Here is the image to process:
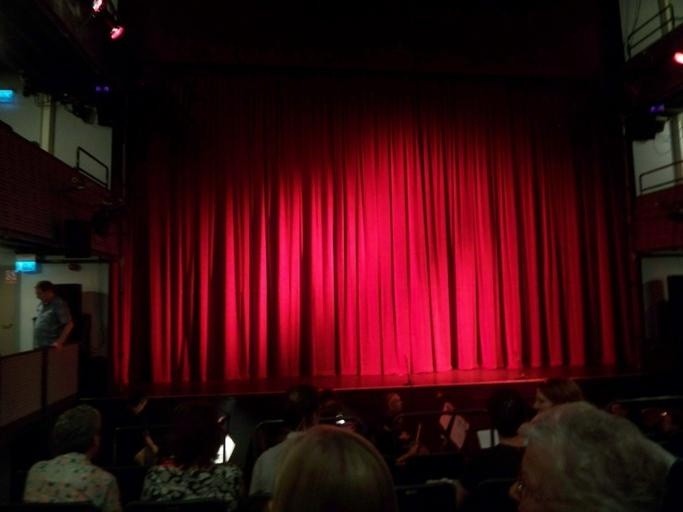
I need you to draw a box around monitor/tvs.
[211,432,238,464]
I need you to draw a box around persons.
[15,377,683,510]
[31,280,75,351]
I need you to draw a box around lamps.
[616,93,682,143]
[81,0,123,40]
